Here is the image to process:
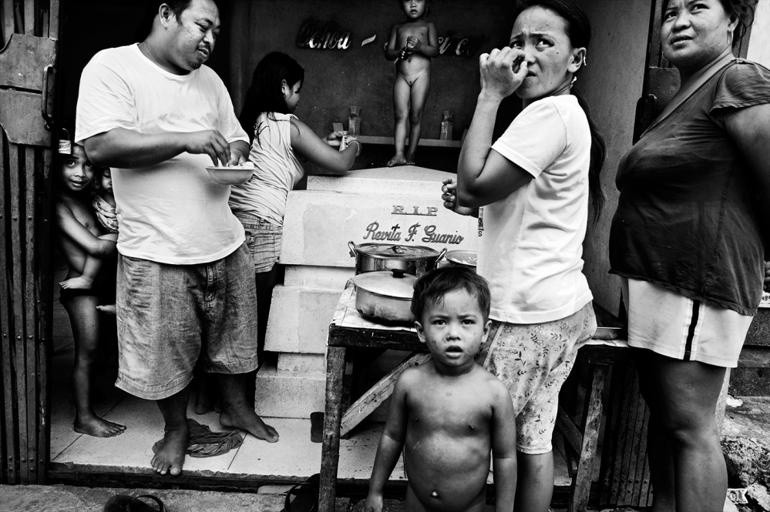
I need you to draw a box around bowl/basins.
[209,165,253,182]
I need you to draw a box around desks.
[318,279,635,510]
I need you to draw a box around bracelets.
[347,140,361,157]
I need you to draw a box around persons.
[382,0,442,167]
[57,165,119,315]
[73,0,279,473]
[363,262,518,512]
[439,2,597,511]
[191,48,363,416]
[53,137,128,440]
[606,1,770,512]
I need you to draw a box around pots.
[352,272,429,324]
[348,234,443,275]
